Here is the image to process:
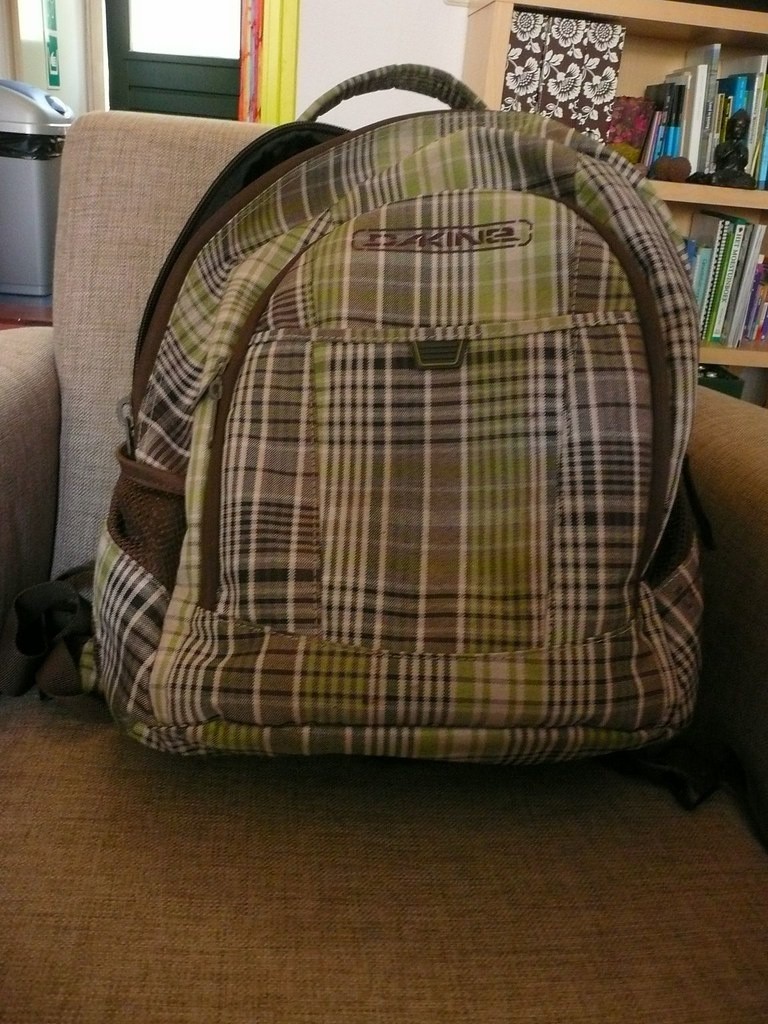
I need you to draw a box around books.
[638,44,768,189]
[681,209,768,349]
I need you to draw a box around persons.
[710,108,756,188]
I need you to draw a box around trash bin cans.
[0,76,73,297]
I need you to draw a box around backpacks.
[80,63,707,770]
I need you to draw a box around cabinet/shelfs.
[462,0,768,410]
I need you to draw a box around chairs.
[0,108,768,1022]
